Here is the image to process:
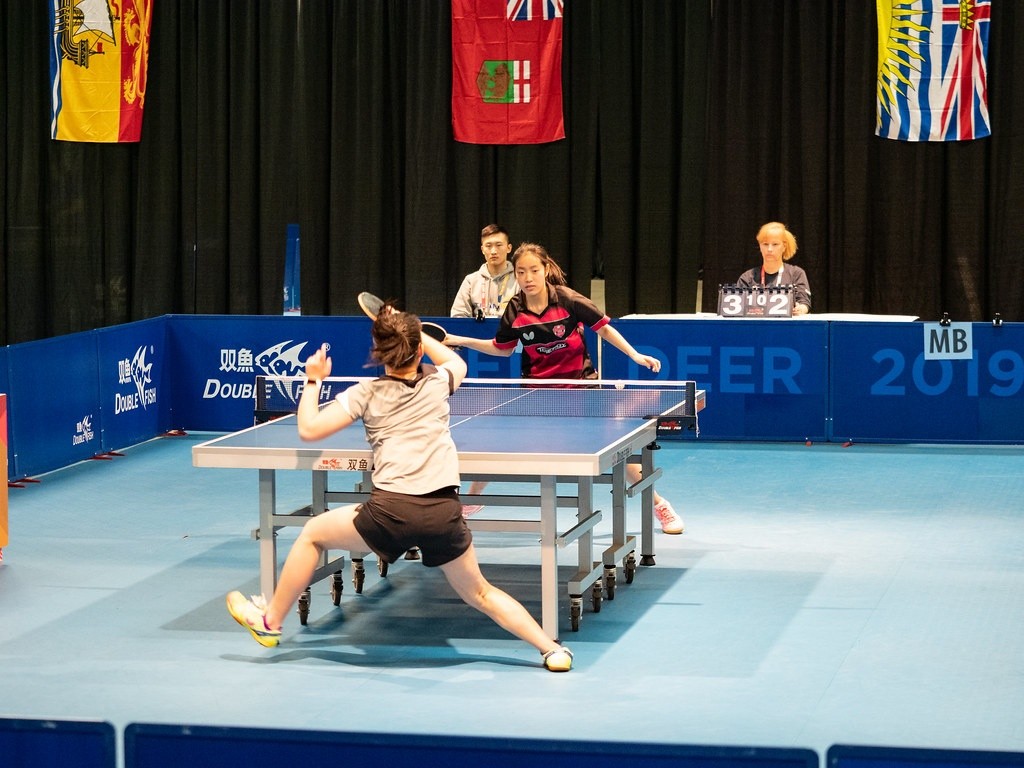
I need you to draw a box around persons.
[443,242,685,534]
[226,306,574,673]
[450,226,523,319]
[737,223,812,314]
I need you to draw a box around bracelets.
[303,378,322,388]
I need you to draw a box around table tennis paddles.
[358,292,384,320]
[420,321,463,350]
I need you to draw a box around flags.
[451,0,567,145]
[46,0,151,143]
[877,0,991,141]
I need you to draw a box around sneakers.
[541,648,573,670]
[654,497,684,533]
[461,504,483,518]
[226,590,282,648]
